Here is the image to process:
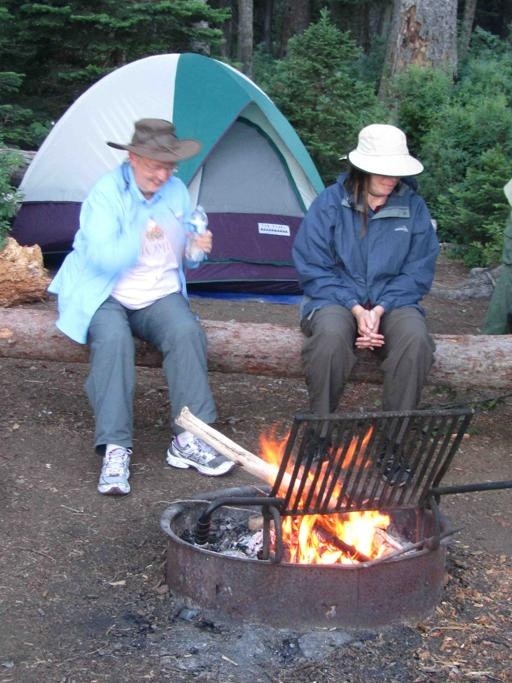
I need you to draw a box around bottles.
[185,202,208,262]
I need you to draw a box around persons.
[292,124,440,489]
[47,117,236,497]
[484,178,512,338]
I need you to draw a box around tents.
[12,52,324,306]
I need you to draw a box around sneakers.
[296,435,412,487]
[96,448,134,496]
[165,434,235,477]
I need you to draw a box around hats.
[337,123,425,178]
[107,118,201,164]
